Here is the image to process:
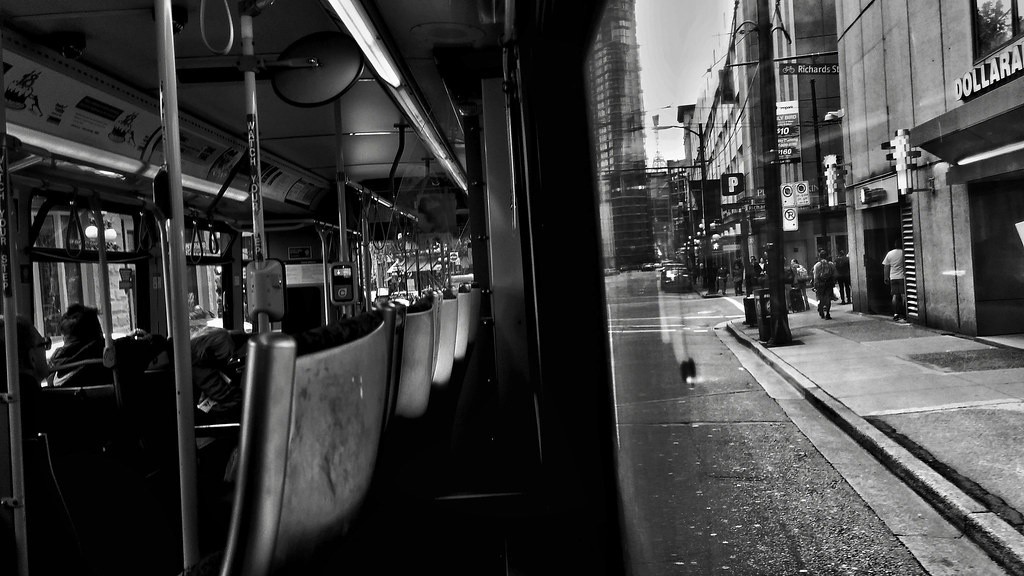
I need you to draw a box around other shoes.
[826,314,832,319]
[848,301,851,303]
[842,301,845,304]
[894,315,900,321]
[821,314,825,318]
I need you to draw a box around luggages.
[790,284,805,313]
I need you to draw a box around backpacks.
[820,261,833,282]
[835,257,849,273]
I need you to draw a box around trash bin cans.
[742,297,758,325]
[752,288,772,342]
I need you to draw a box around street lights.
[651,173,696,284]
[656,125,716,294]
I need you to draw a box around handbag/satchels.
[796,265,808,280]
[734,274,742,282]
[765,274,768,280]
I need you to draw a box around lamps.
[894,129,935,197]
[824,163,847,207]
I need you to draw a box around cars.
[654,261,692,293]
[605,262,656,275]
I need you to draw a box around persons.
[0,314,50,397]
[881,239,904,321]
[187,328,248,485]
[712,246,851,321]
[43,305,106,391]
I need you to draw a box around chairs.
[0,283,483,576]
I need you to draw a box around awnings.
[909,76,1024,164]
